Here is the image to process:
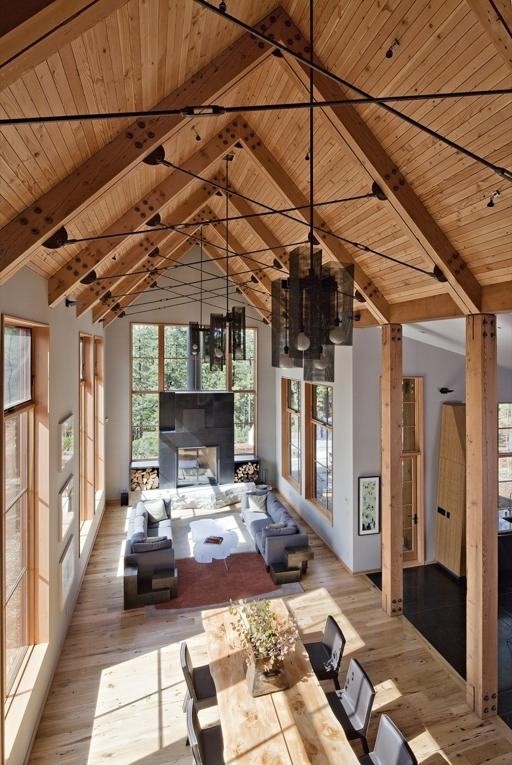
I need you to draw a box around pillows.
[247,494,267,513]
[145,500,168,525]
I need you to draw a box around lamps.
[272,279,303,368]
[209,313,223,372]
[289,246,320,359]
[203,324,211,364]
[231,306,246,360]
[320,260,355,346]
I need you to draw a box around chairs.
[304,616,346,691]
[326,658,376,753]
[357,714,416,765]
[179,642,217,713]
[184,699,224,765]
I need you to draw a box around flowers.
[223,601,299,667]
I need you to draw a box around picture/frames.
[357,475,382,537]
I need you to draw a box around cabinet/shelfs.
[159,390,235,488]
[401,376,424,455]
[434,403,467,578]
[401,458,426,569]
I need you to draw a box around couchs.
[124,502,176,573]
[240,490,310,573]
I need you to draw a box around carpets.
[155,552,275,609]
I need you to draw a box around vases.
[245,654,290,697]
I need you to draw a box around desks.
[202,601,360,765]
